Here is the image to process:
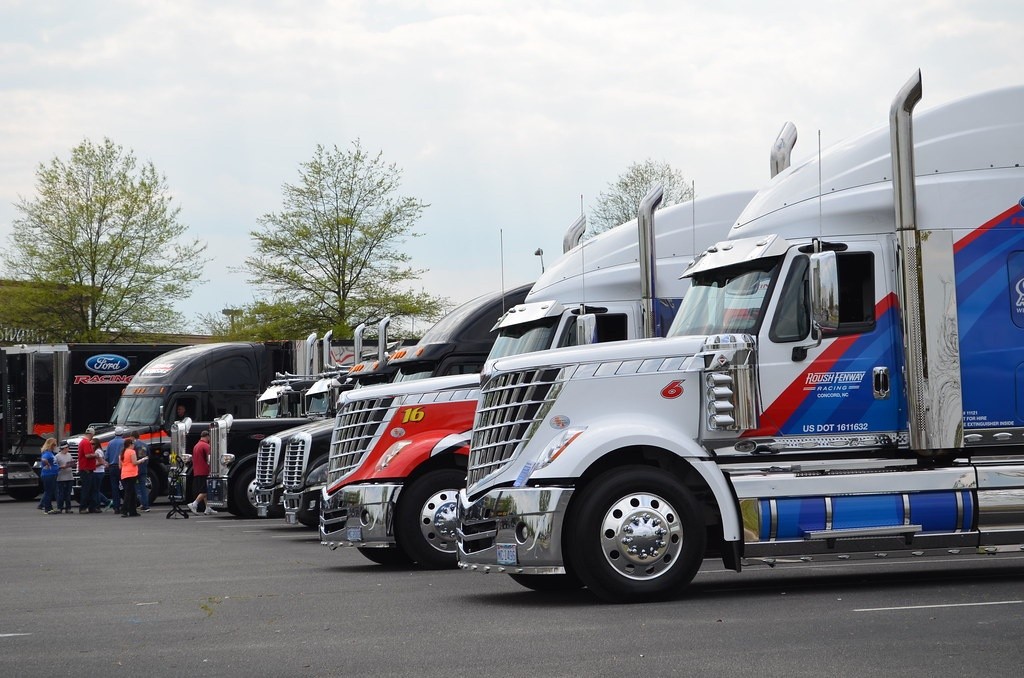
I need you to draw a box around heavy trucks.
[168,327,426,517]
[33,338,431,513]
[244,67,1024,603]
[0,343,193,504]
[206,280,539,522]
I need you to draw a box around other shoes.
[121,512,129,517]
[35,505,45,511]
[44,510,50,514]
[79,507,88,513]
[141,506,151,512]
[129,513,141,517]
[65,508,73,514]
[88,508,102,513]
[104,500,113,511]
[137,505,143,509]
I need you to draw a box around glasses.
[91,443,95,445]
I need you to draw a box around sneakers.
[204,509,217,515]
[188,503,199,515]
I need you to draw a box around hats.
[86,428,95,434]
[59,440,69,448]
[115,427,124,435]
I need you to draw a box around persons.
[187,430,218,515]
[38,428,150,518]
[175,406,185,420]
